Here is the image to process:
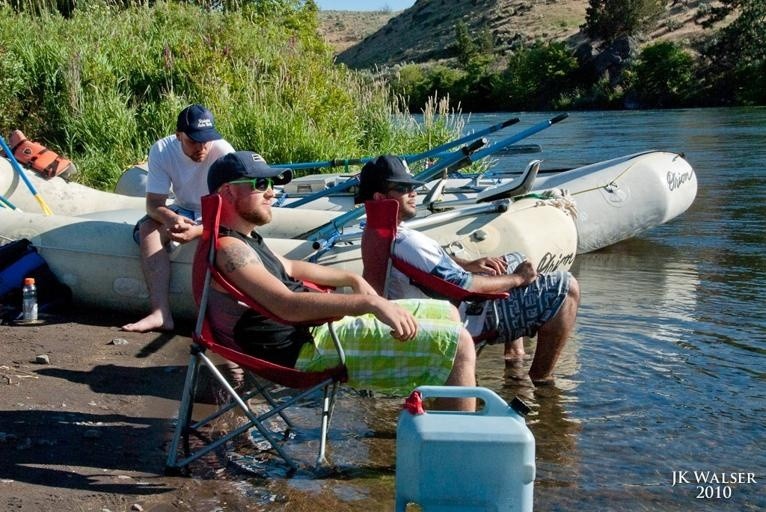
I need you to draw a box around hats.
[358,154,426,191]
[206,151,293,195]
[175,104,222,143]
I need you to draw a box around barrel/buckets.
[396,385,536,512]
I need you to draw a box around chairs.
[167,192,345,467]
[362,198,511,302]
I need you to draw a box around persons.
[200,149,479,412]
[120,102,238,333]
[355,152,584,384]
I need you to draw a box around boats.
[0,149,698,318]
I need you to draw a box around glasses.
[218,177,276,192]
[391,187,419,193]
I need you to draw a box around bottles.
[23,278,39,321]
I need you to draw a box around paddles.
[0,137,53,216]
